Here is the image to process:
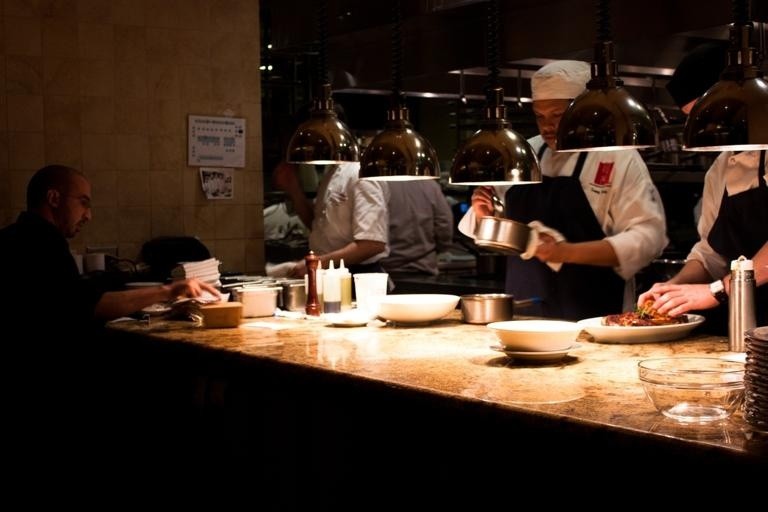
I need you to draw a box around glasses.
[64,195,93,209]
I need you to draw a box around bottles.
[305,251,352,316]
[728,255,758,354]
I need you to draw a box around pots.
[456,294,542,325]
[475,215,532,258]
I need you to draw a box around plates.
[577,313,706,344]
[744,326,767,428]
[140,307,172,317]
[488,343,583,363]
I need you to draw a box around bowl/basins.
[370,293,461,324]
[487,320,583,350]
[638,358,746,422]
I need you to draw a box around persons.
[205,172,232,197]
[262,57,768,336]
[1,164,221,511]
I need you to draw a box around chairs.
[138,235,211,263]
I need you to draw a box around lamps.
[287,1,362,166]
[680,2,767,154]
[446,1,543,186]
[554,0,656,154]
[357,0,441,182]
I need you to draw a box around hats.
[531,60,591,103]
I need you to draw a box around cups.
[355,272,389,314]
